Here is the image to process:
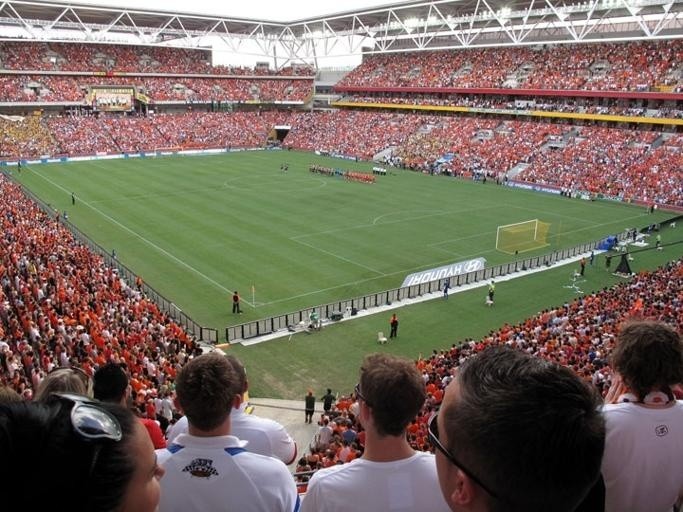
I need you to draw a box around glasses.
[353,384,374,411]
[50,394,121,472]
[425,412,495,502]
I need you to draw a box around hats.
[92,361,128,395]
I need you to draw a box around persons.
[166,355,299,465]
[591,321,682,512]
[0,386,165,510]
[1,35,683,215]
[232,290,240,313]
[0,181,203,451]
[427,348,606,511]
[154,351,300,512]
[294,351,452,511]
[294,224,683,490]
